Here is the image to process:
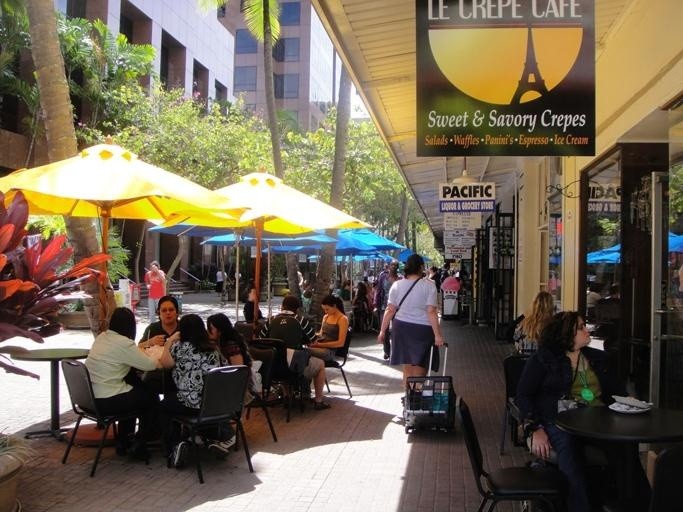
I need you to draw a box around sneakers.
[168,440,189,468]
[128,442,150,460]
[207,441,230,459]
[220,434,238,448]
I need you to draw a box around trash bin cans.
[169,292,183,312]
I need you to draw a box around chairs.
[311,327,351,400]
[458,354,683,512]
[252,338,305,424]
[233,344,279,449]
[594,301,620,329]
[61,359,149,477]
[169,369,249,484]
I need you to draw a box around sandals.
[315,400,330,409]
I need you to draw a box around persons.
[511,311,651,512]
[432,263,468,318]
[548,272,561,300]
[146,260,401,409]
[587,275,620,324]
[379,254,444,420]
[85,297,248,466]
[514,290,560,350]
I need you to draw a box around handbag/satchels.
[555,392,588,413]
[519,456,563,511]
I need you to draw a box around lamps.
[453,156,480,183]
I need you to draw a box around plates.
[608,395,652,414]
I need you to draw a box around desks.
[10,348,90,442]
